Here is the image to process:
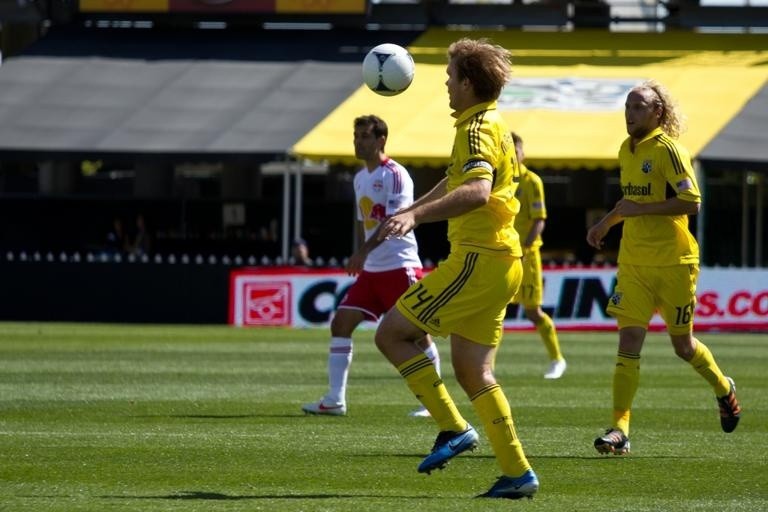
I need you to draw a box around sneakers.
[408,401,432,418]
[301,395,348,416]
[716,376,741,433]
[543,358,567,379]
[470,468,540,501]
[594,427,631,456]
[417,422,480,476]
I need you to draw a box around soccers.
[361,42,414,96]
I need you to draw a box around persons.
[375,36,540,500]
[511,132,566,379]
[587,85,740,454]
[304,116,441,417]
